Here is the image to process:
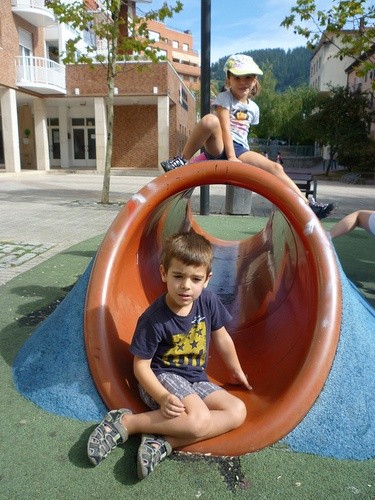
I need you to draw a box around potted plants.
[23,129,31,144]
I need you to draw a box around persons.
[87,233,254,480]
[327,209,375,238]
[160,54,335,221]
[260,151,284,165]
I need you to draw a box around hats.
[222,53,264,78]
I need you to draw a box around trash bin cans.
[225,186,252,215]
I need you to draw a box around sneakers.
[308,197,335,220]
[160,156,189,172]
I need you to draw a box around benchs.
[284,168,317,201]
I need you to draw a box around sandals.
[137,432,173,479]
[88,408,132,466]
